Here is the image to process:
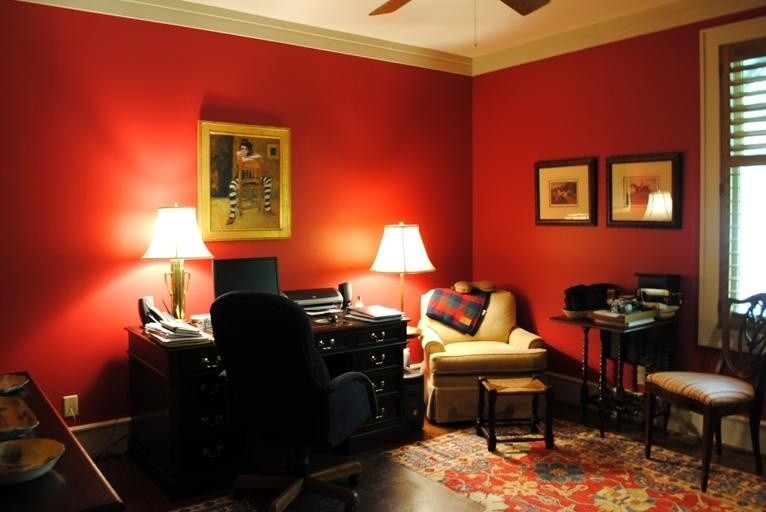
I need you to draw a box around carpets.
[175,415,766,512]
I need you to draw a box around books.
[144,317,215,347]
[345,304,404,323]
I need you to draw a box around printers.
[281,287,344,316]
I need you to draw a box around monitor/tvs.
[212,256,279,299]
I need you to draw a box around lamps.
[370,222,435,314]
[140,204,214,318]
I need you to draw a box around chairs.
[644,293,766,492]
[210,289,379,512]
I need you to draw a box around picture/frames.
[534,157,597,226]
[605,152,682,229]
[196,120,292,243]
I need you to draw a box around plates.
[0,438,65,481]
[0,374,30,393]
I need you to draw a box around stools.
[475,372,553,451]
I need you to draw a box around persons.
[223,136,274,226]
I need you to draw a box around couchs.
[417,288,548,422]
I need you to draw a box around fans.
[367,0,549,16]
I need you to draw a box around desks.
[124,307,411,502]
[0,371,121,512]
[548,315,681,439]
